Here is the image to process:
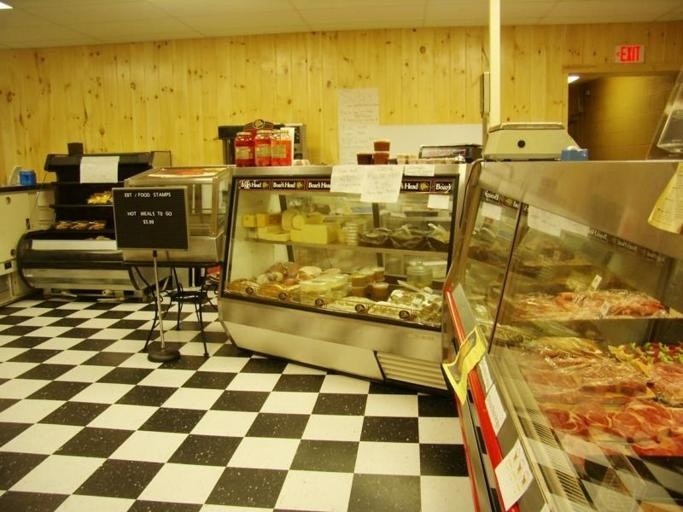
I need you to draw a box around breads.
[228,259,341,304]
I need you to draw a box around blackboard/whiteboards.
[112,186,189,251]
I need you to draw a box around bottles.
[233,128,293,167]
[405,258,433,289]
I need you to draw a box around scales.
[481,122,580,161]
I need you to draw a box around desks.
[124,257,224,357]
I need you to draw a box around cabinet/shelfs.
[215,163,472,393]
[16,149,174,304]
[436,153,682,512]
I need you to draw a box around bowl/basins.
[358,230,449,250]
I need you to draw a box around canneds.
[233,130,292,167]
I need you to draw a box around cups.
[18,170,35,186]
